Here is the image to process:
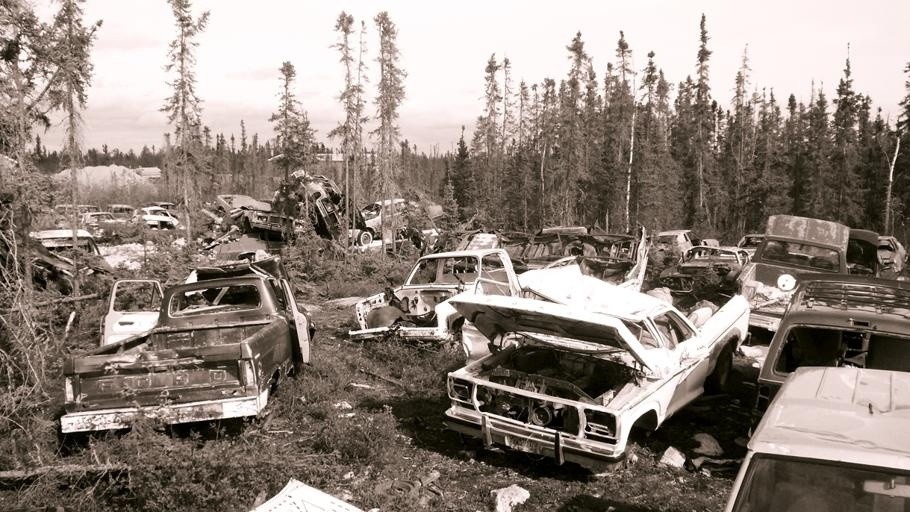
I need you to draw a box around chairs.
[765,245,788,261]
[810,255,832,271]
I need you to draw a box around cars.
[347,212,910,478]
[23,171,444,296]
[720,361,910,510]
[60,249,318,433]
